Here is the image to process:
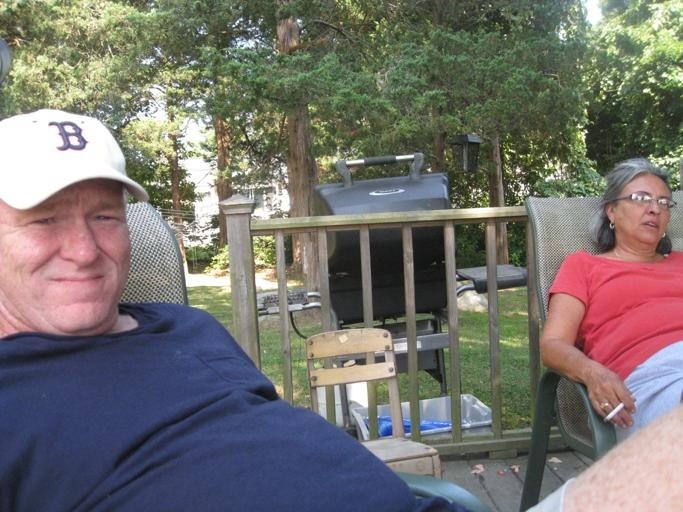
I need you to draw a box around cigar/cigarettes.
[601,401,625,424]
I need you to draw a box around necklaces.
[611,246,662,264]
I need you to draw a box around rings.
[597,401,610,411]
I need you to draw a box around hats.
[1,108,151,212]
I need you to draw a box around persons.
[538,156,682,446]
[0,108,682,512]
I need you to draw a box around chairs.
[305,328,442,480]
[518,191,683,512]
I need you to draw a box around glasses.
[606,193,676,209]
